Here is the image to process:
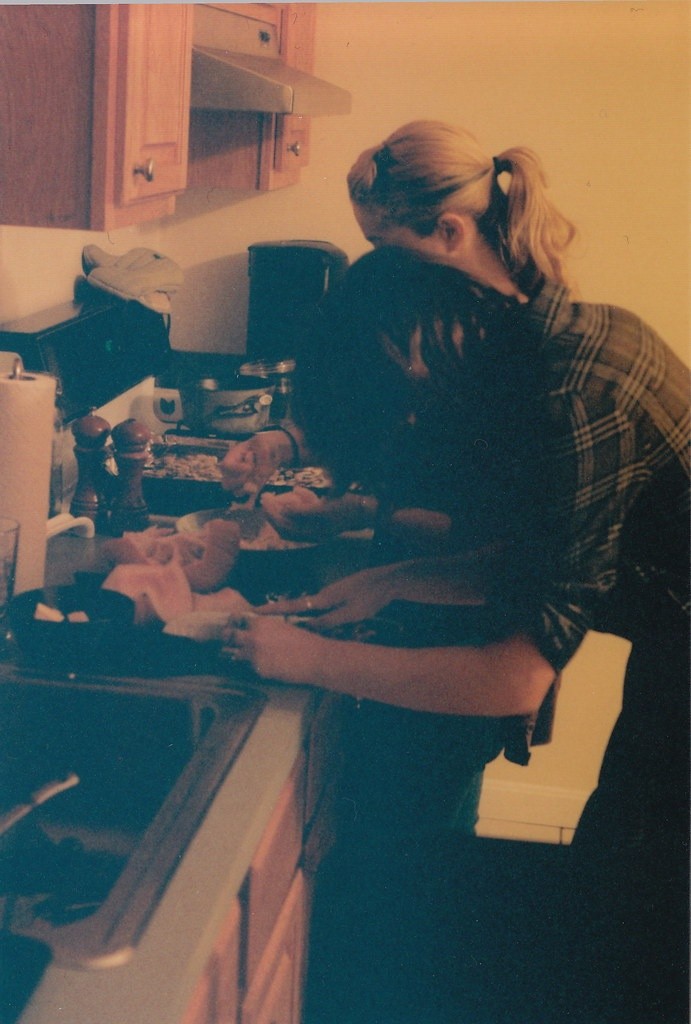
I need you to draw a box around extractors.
[190,4,352,115]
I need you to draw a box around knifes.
[161,610,405,646]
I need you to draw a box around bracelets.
[260,425,301,469]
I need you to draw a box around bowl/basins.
[175,508,345,580]
[241,360,299,396]
[7,584,136,668]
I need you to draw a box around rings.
[305,598,313,609]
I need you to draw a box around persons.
[219,121,690,1024]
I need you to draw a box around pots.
[183,370,277,434]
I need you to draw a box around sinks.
[0,672,271,970]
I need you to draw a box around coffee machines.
[246,239,350,356]
